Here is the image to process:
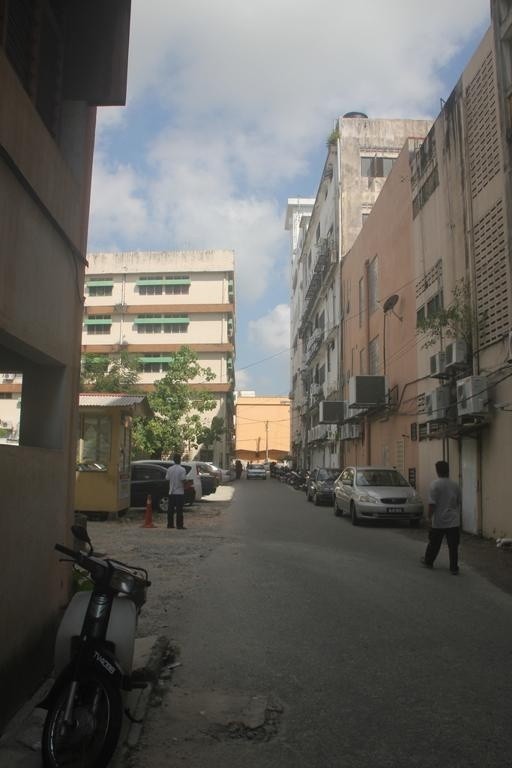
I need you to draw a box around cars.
[262,461,281,472]
[304,463,345,507]
[332,464,424,527]
[129,455,237,514]
[247,461,269,481]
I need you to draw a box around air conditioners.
[444,343,466,368]
[430,352,452,377]
[319,401,343,424]
[417,388,450,425]
[313,329,322,337]
[348,376,384,408]
[311,384,321,396]
[456,375,488,417]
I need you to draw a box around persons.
[165,455,188,529]
[421,461,463,575]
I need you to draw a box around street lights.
[264,418,273,462]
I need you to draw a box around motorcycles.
[34,516,161,767]
[272,463,313,494]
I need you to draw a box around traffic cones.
[140,494,157,528]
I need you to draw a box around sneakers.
[420,555,435,568]
[166,524,187,529]
[450,565,461,575]
